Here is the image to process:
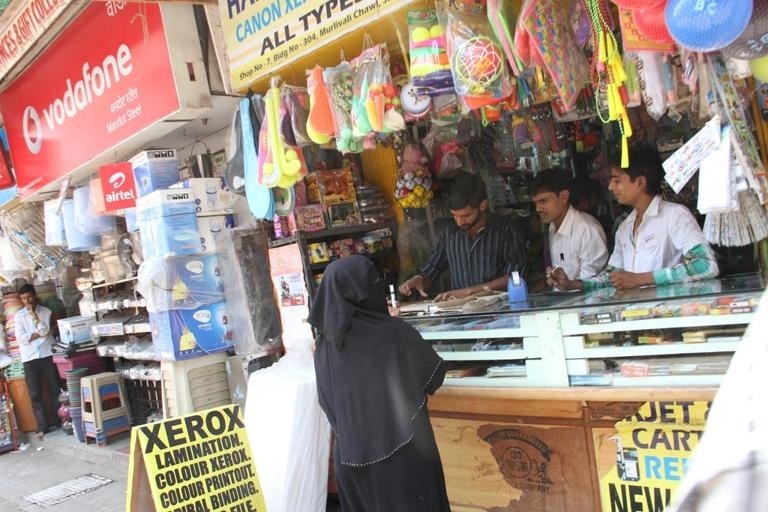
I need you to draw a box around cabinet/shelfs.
[91,275,161,422]
[268,167,764,512]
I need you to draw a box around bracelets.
[412,275,427,280]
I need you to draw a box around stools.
[80,371,135,447]
[161,351,233,420]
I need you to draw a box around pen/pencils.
[389,284,396,308]
[546,259,563,277]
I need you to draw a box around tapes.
[511,271,520,287]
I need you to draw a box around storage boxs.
[57,315,96,346]
[137,179,235,358]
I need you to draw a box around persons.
[171,274,191,304]
[399,170,527,304]
[541,143,721,293]
[179,325,197,352]
[304,253,457,512]
[14,283,65,441]
[530,166,610,293]
